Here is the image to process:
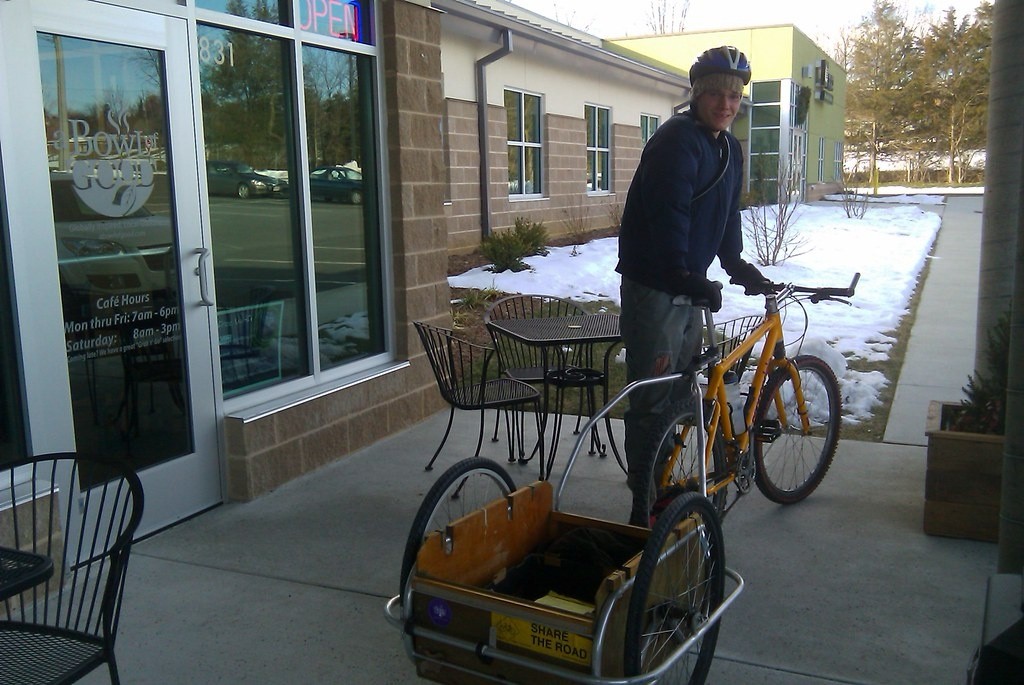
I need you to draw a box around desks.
[485,313,629,480]
[0,545,53,603]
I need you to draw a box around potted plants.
[923,295,1014,543]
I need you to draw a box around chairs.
[412,320,545,503]
[61,283,277,459]
[484,295,594,464]
[676,314,764,426]
[0,453,145,685]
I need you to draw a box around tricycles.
[384,272,861,685]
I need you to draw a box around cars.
[52,171,178,329]
[310,166,363,204]
[205,160,284,199]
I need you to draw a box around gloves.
[688,275,723,312]
[730,264,770,295]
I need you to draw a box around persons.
[614,46,769,529]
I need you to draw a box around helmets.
[689,46,752,87]
